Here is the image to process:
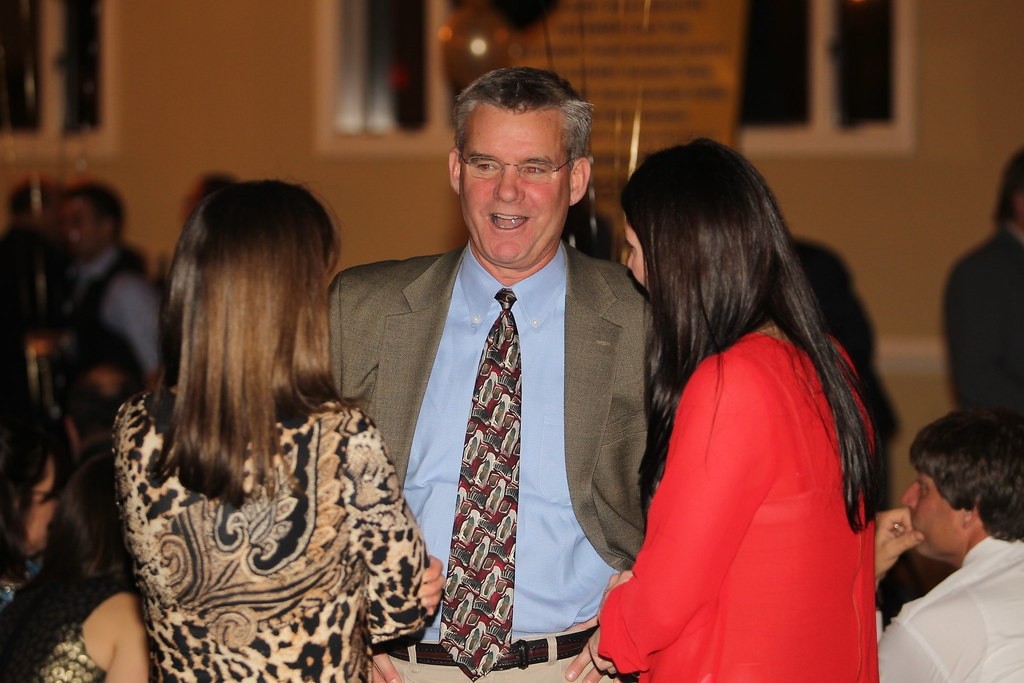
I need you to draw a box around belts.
[384,627,600,671]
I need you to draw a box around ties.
[439,288,523,680]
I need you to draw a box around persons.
[0,171,235,683]
[790,142,1024,683]
[329,65,654,683]
[589,136,880,683]
[111,178,446,683]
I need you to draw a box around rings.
[889,523,901,532]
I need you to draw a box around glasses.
[459,152,577,185]
[27,487,56,505]
